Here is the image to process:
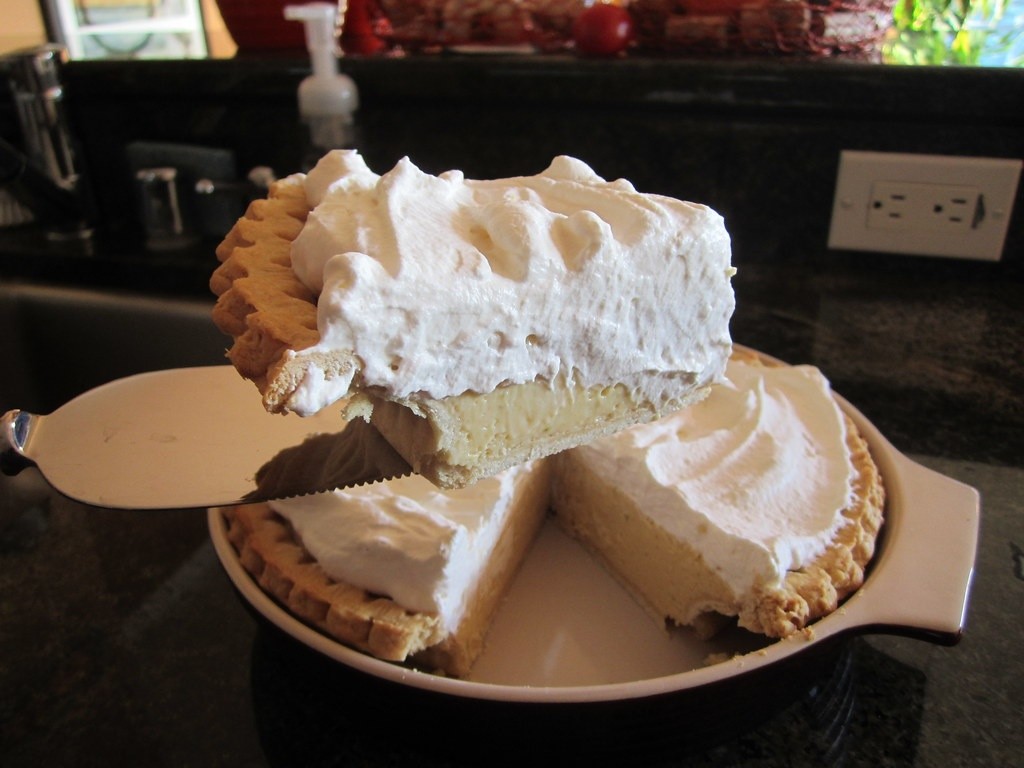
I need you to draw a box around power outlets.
[827,151,1023,262]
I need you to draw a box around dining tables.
[0,446,1024,768]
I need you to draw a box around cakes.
[213,147,739,480]
[221,346,889,679]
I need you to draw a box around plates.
[206,339,981,705]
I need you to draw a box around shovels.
[0,363,433,511]
